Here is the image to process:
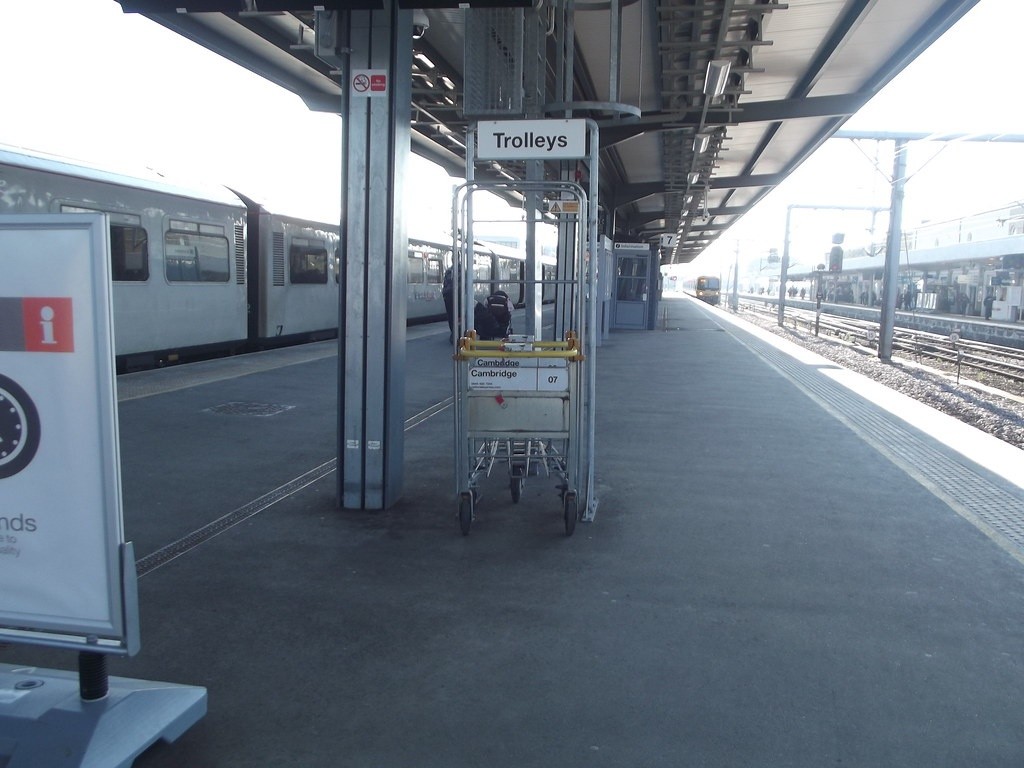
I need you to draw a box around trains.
[0,148,556,374]
[683,275,721,305]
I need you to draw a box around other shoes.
[449,338,453,344]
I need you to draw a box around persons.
[958,293,970,316]
[800,288,805,299]
[823,289,827,300]
[897,289,917,310]
[758,287,770,295]
[443,254,467,344]
[860,291,876,306]
[788,286,798,297]
[474,291,514,338]
[750,287,754,293]
[984,294,993,320]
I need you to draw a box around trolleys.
[457,328,584,536]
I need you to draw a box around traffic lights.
[828,246,842,272]
